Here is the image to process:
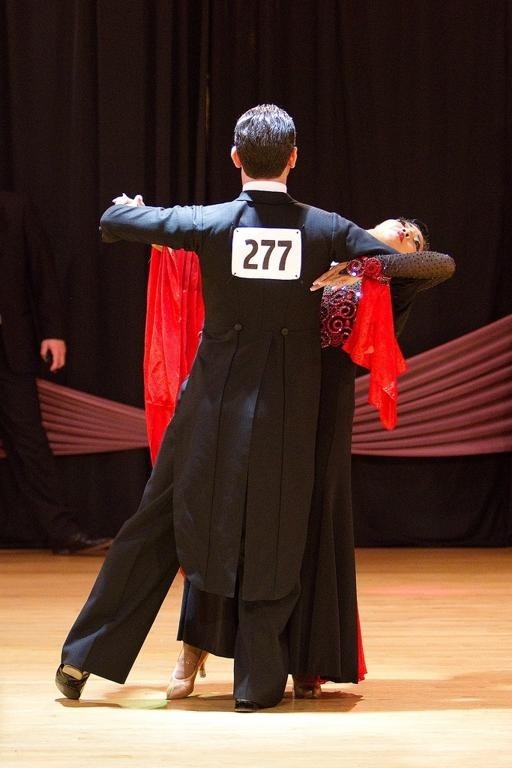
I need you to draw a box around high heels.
[166,647,210,699]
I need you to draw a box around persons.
[55,105,405,712]
[114,194,456,700]
[0,190,113,555]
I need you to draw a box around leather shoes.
[56,532,115,555]
[289,677,329,700]
[53,659,89,701]
[231,692,266,711]
